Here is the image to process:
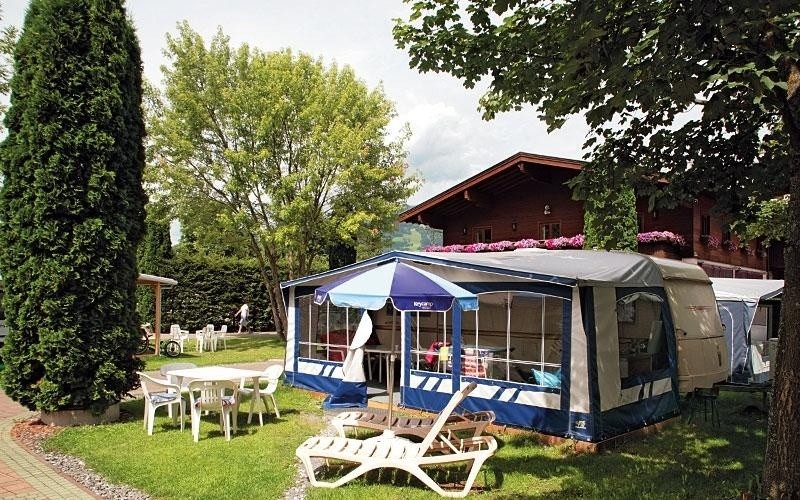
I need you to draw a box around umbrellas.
[312,257,479,432]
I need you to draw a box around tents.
[710,278,784,385]
[280,250,729,443]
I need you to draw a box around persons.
[234,301,251,334]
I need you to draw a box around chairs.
[170,324,190,353]
[294,381,499,499]
[136,359,285,443]
[196,323,228,353]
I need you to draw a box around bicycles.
[134,329,181,357]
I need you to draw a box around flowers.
[700,234,756,257]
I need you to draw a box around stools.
[687,386,721,430]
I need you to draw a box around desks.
[364,344,514,394]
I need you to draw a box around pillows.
[530,367,561,387]
